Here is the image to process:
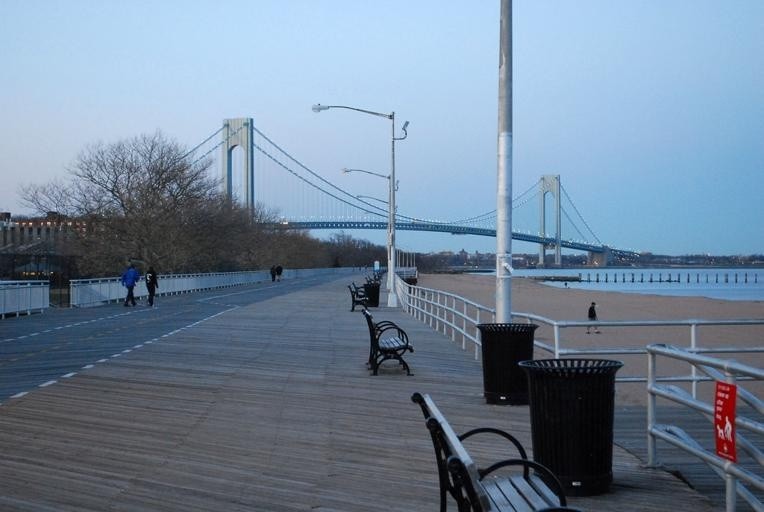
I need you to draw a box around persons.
[270,264,276,283]
[145,266,159,306]
[121,264,141,307]
[275,264,283,282]
[585,301,601,334]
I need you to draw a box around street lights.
[309,102,411,307]
[342,166,397,288]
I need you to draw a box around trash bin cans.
[475,323,540,404]
[518,358,624,497]
[364,283,381,306]
[405,278,417,293]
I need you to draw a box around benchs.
[361,309,414,376]
[347,282,369,312]
[411,392,586,512]
[365,270,386,284]
